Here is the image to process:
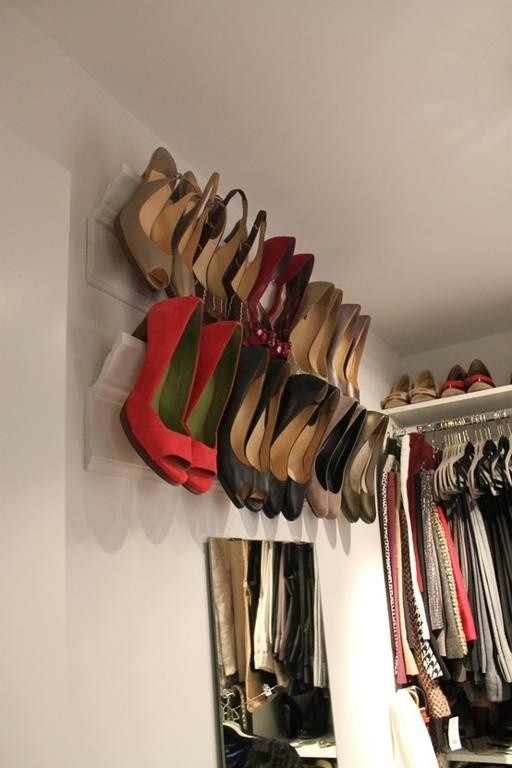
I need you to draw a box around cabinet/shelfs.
[86,165,339,498]
[373,384,511,768]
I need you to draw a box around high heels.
[298,690,330,740]
[111,146,390,525]
[281,693,299,738]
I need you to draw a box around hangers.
[432,409,511,503]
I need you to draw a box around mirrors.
[205,537,338,768]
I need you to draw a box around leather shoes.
[440,363,467,396]
[410,369,439,403]
[383,373,412,407]
[466,358,496,391]
